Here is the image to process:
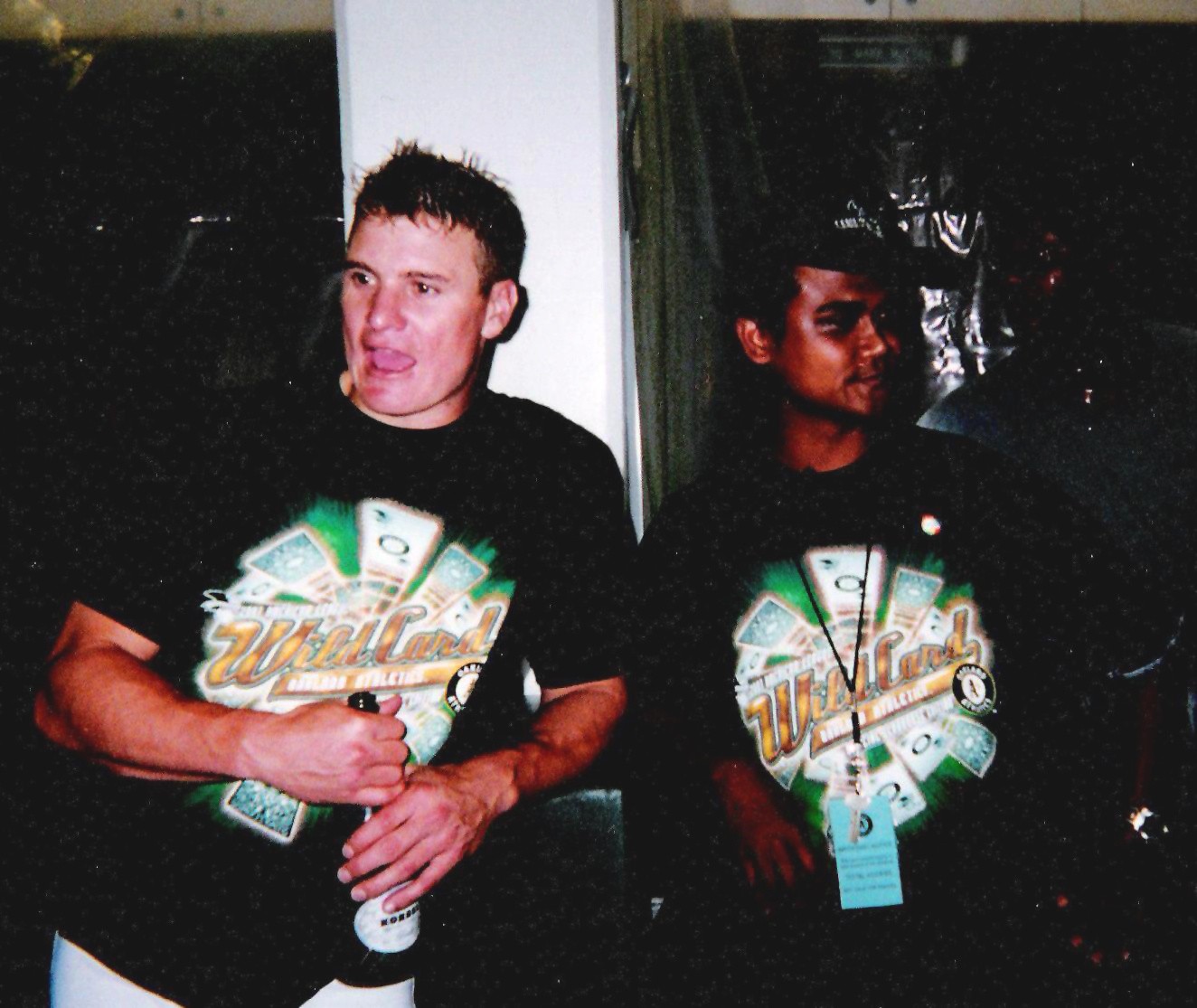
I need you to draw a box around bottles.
[328,692,428,986]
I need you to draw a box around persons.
[33,133,639,1008]
[639,223,1045,1008]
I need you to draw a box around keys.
[842,791,870,843]
